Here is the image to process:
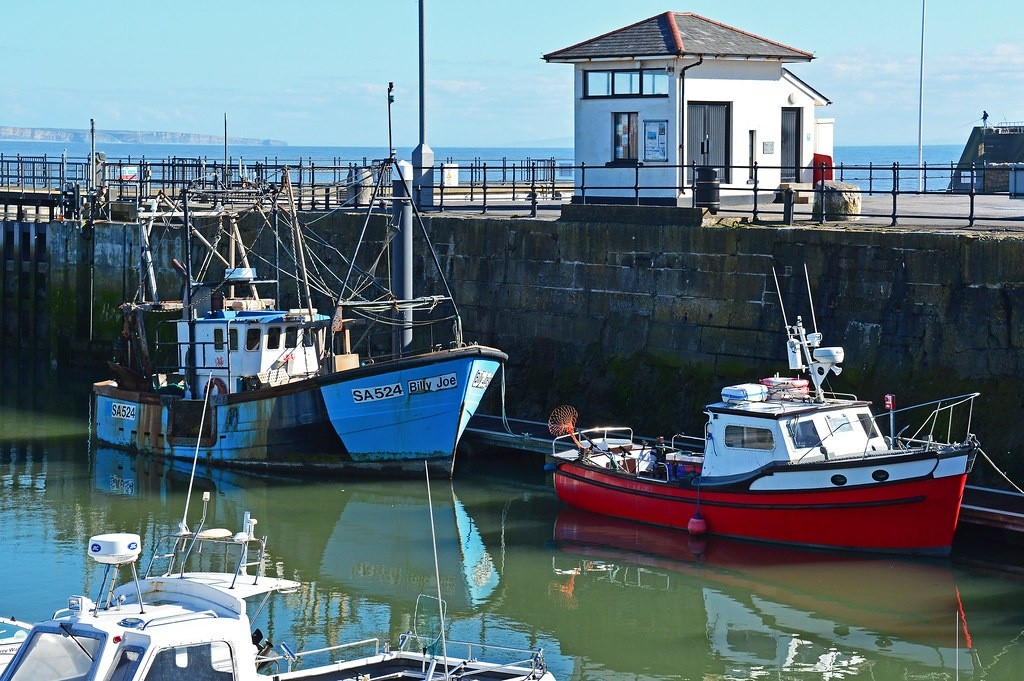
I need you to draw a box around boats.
[1,512,564,681]
[91,263,513,486]
[548,327,978,567]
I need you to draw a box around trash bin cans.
[694,166,720,216]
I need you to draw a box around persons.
[981,109,990,129]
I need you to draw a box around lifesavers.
[203,378,227,400]
[763,377,809,388]
[768,387,810,400]
